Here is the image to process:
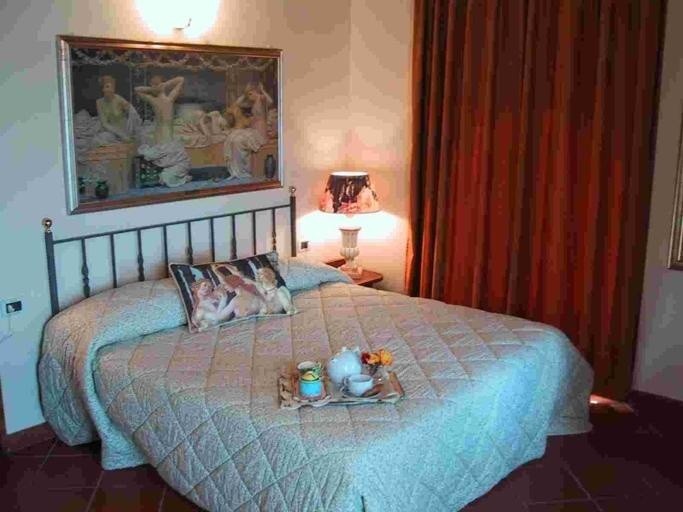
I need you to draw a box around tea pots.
[325,345,364,387]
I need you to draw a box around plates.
[340,385,382,399]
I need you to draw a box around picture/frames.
[55,34,284,215]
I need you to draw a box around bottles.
[299,373,321,397]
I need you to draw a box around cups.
[297,360,320,372]
[342,374,372,396]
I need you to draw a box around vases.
[361,367,389,386]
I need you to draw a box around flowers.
[357,347,393,376]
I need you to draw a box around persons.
[96,74,272,187]
[191,264,291,330]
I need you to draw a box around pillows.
[168,250,302,335]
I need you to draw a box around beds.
[42,184,568,512]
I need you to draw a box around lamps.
[317,171,384,279]
